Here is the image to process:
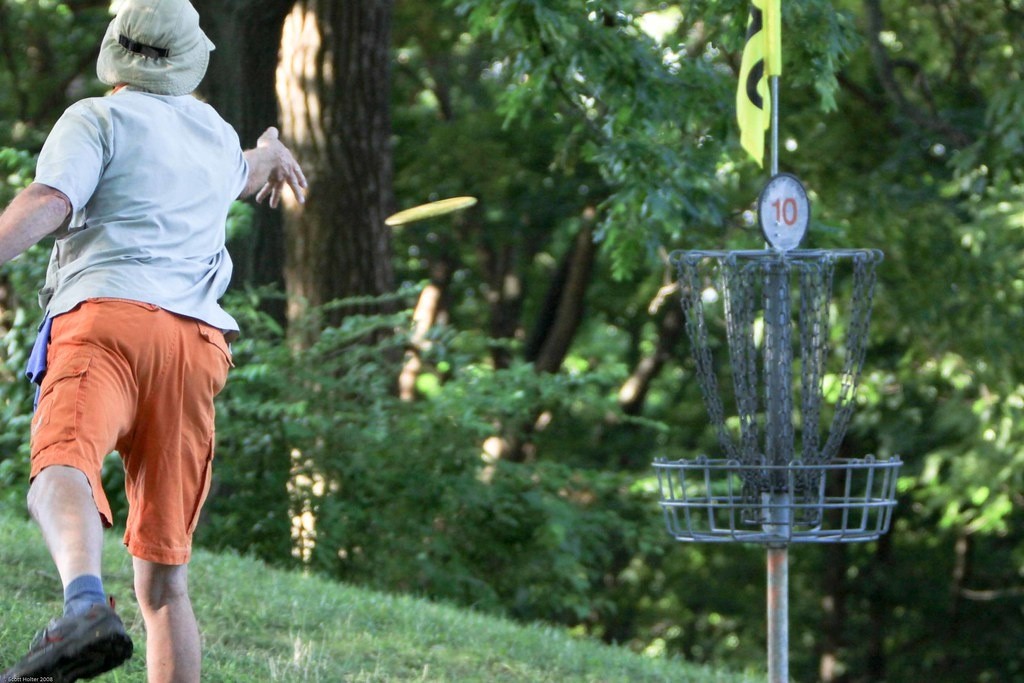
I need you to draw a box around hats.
[96,0,217,97]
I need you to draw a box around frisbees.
[385,196,478,226]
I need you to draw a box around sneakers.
[0,595,133,683]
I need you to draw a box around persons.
[0,0,307,683]
